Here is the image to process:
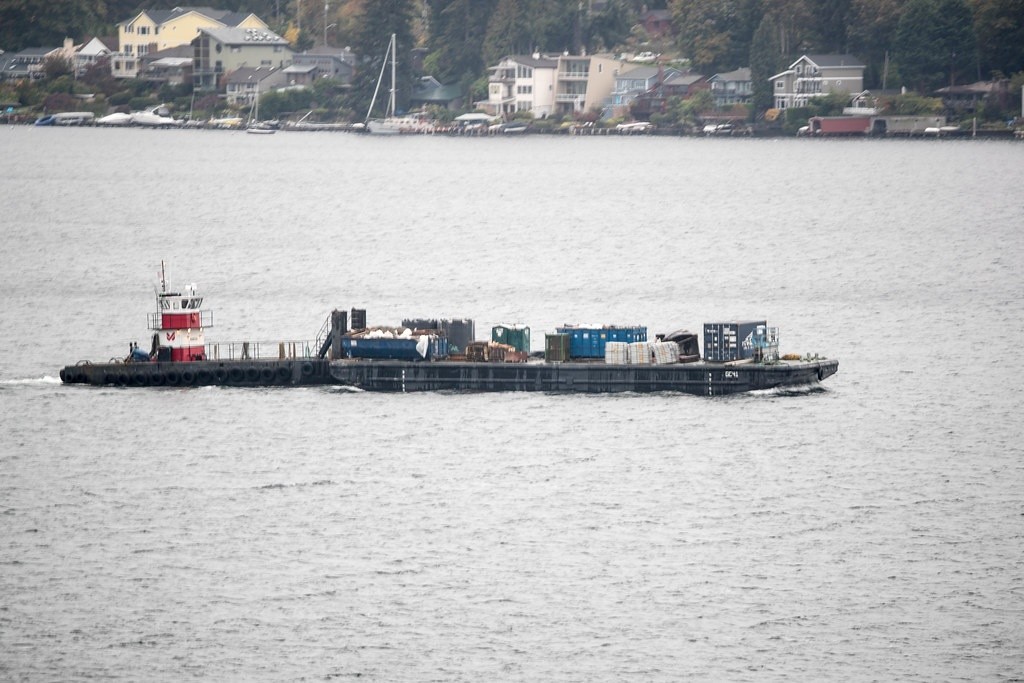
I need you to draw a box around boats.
[327,319,841,396]
[96,111,130,126]
[206,118,244,130]
[59,261,346,388]
[284,121,349,131]
[34,116,53,126]
[51,112,94,126]
[247,122,272,130]
[128,104,177,126]
[185,120,205,128]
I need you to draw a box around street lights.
[973,117,977,138]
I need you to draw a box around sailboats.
[366,33,436,134]
[246,79,276,134]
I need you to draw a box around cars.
[703,124,732,134]
[616,120,650,131]
